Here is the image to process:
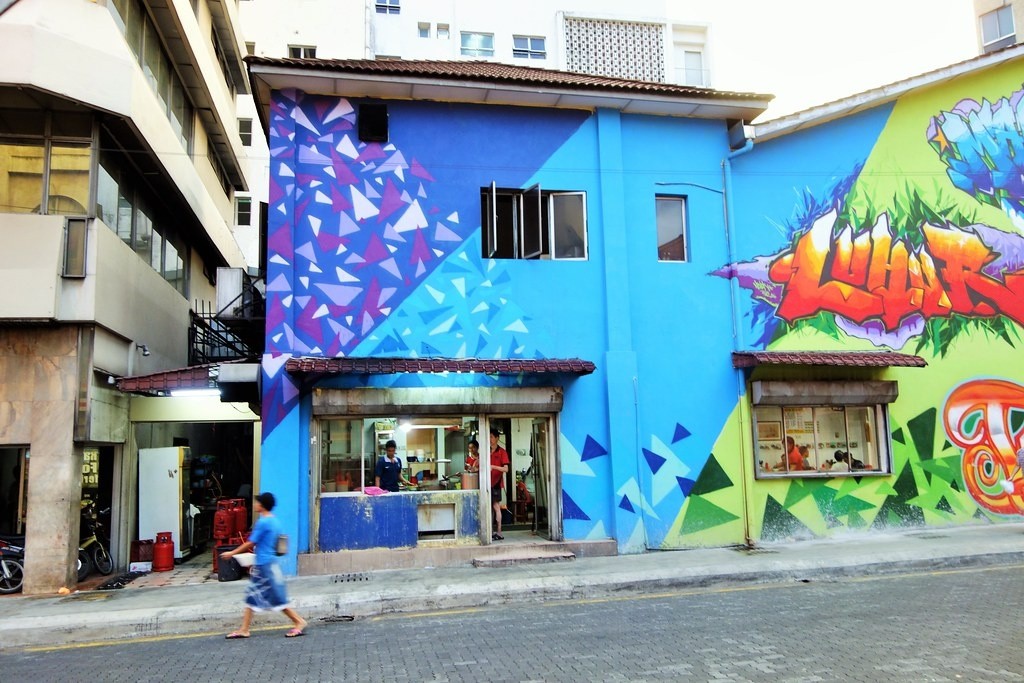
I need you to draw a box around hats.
[254,492,275,512]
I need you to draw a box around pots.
[462,471,478,489]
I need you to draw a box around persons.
[843,451,865,469]
[219,492,308,639]
[375,440,415,493]
[829,450,849,473]
[779,454,786,467]
[464,439,480,489]
[798,445,811,470]
[490,427,510,541]
[773,436,803,472]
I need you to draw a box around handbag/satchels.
[275,535,287,556]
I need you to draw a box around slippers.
[225,632,249,639]
[492,533,504,541]
[284,628,305,637]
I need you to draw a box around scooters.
[0,497,114,595]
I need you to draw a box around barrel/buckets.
[216,545,241,582]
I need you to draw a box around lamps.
[135,342,150,357]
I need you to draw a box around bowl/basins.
[418,458,424,462]
[407,457,415,462]
